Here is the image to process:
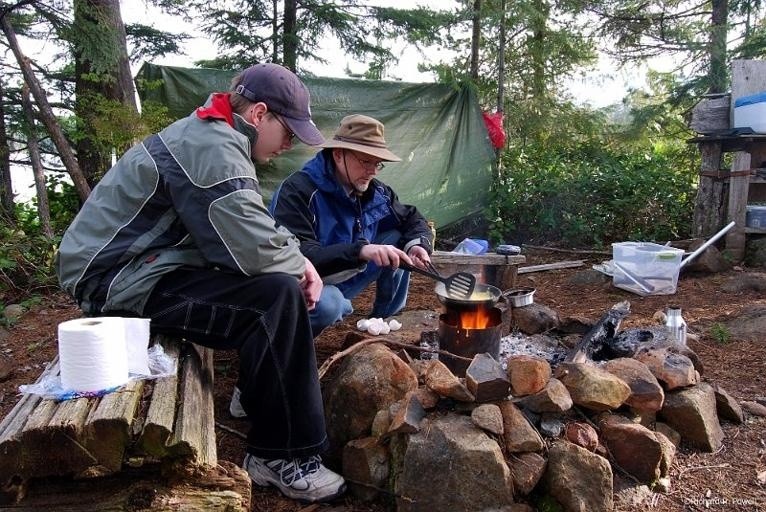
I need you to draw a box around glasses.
[348,150,385,171]
[267,110,296,139]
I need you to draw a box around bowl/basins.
[502,286,537,307]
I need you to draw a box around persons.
[59,63,348,504]
[268,115,434,338]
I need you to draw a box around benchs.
[430,252,527,292]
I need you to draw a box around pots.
[434,282,502,324]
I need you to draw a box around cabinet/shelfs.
[685,60,766,263]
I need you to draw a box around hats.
[235,62,326,146]
[314,114,403,163]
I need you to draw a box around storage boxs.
[747,207,766,228]
[612,241,685,296]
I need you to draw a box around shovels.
[390,259,476,300]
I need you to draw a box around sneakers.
[230,386,247,419]
[242,454,348,502]
